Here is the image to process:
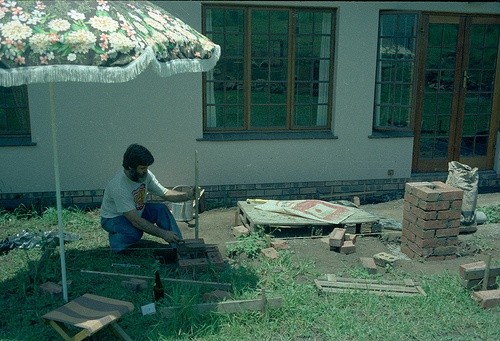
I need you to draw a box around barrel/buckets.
[172,185,193,221]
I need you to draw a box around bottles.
[153,271,164,300]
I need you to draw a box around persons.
[101,143,202,254]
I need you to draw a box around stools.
[41,293,135,341]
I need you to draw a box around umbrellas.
[381,44,415,58]
[0,0,222,302]
